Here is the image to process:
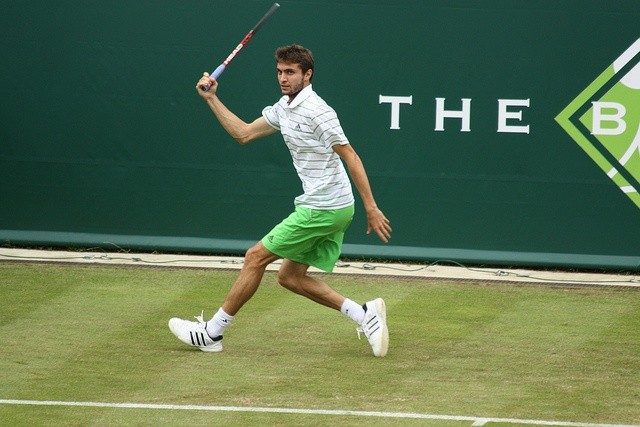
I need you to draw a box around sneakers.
[168,309,224,352]
[356,298,390,357]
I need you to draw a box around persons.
[167,45,393,358]
